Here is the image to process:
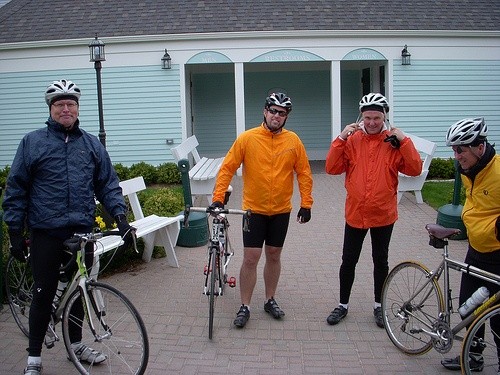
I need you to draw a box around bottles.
[218,241,225,256]
[218,230,225,249]
[457,287,490,317]
[52,271,70,304]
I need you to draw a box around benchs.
[171,135,230,206]
[396,132,437,205]
[83,176,180,280]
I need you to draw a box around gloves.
[115,214,138,254]
[8,227,29,263]
[383,134,398,148]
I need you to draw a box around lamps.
[402,44,411,66]
[161,48,172,68]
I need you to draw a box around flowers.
[94,217,122,233]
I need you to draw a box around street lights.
[402,43,412,66]
[87,46,108,147]
[160,49,172,70]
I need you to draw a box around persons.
[325,92,422,328]
[441,116,500,372]
[2,79,137,375]
[210,93,314,329]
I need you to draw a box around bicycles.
[182,186,252,340]
[380,223,500,375]
[3,225,150,375]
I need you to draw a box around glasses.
[267,106,288,117]
[52,103,78,110]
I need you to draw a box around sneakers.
[24,360,43,375]
[67,342,107,362]
[373,306,384,328]
[441,351,484,371]
[326,305,348,324]
[233,303,250,327]
[264,296,285,319]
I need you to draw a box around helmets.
[266,91,292,109]
[445,117,487,146]
[359,93,389,113]
[44,79,81,106]
